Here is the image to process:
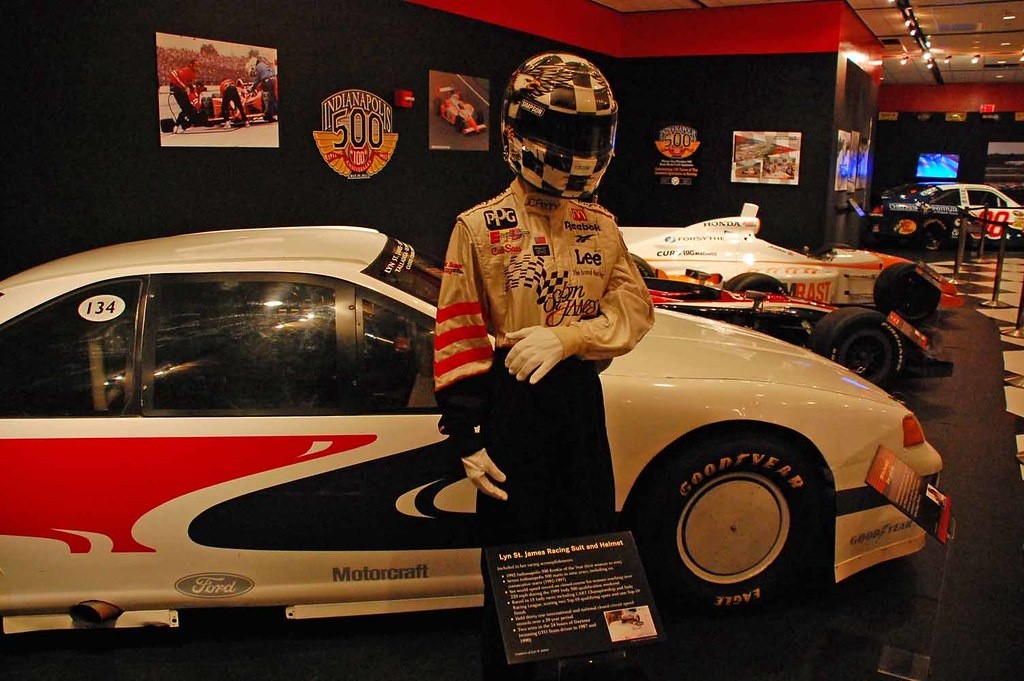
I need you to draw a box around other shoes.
[245,120,250,127]
[173,125,184,133]
[223,121,232,130]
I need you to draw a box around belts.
[265,77,274,82]
[225,84,235,89]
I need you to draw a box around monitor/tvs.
[915,152,960,179]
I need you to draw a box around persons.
[433,50,654,681]
[169,57,277,136]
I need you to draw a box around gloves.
[462,447,508,501]
[504,326,563,385]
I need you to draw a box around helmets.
[501,52,618,199]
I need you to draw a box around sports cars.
[596,204,941,380]
[869,182,1023,251]
[4,226,952,655]
